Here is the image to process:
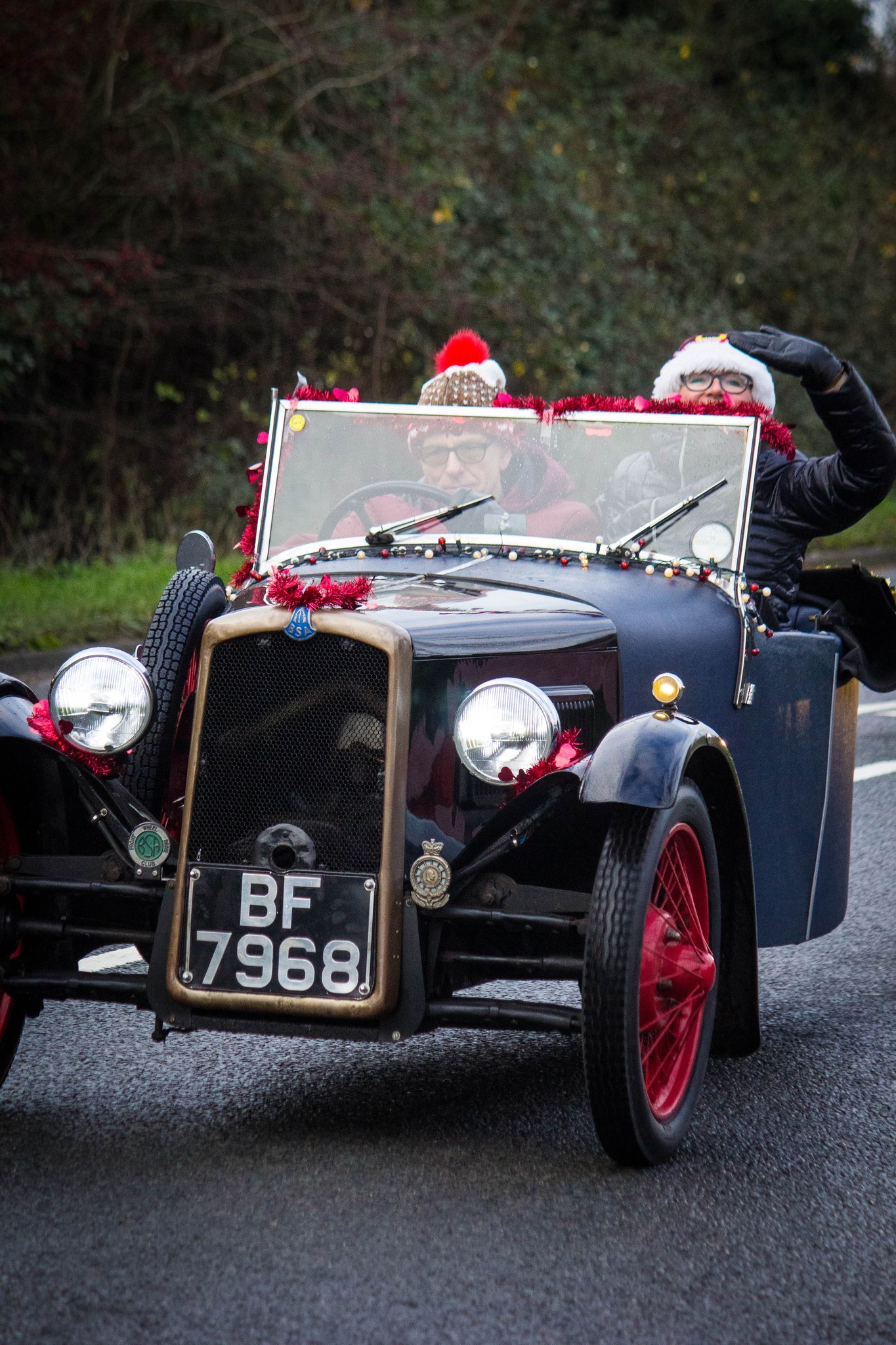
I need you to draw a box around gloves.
[726,325,844,393]
[433,486,506,534]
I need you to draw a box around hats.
[405,329,515,464]
[649,332,776,420]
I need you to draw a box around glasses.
[680,369,754,396]
[414,436,498,467]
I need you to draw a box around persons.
[592,325,895,627]
[266,329,601,543]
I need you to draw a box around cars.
[0,374,860,1175]
